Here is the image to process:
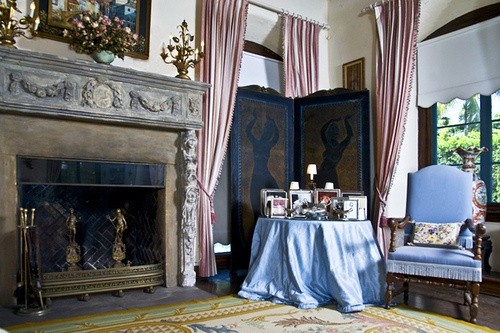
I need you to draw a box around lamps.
[290,164,335,190]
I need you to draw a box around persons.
[66,207,78,245]
[106,207,127,243]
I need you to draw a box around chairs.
[384,165,486,324]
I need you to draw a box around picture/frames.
[260,188,288,218]
[331,191,368,220]
[342,57,365,92]
[290,190,313,218]
[29,0,151,60]
[315,189,342,218]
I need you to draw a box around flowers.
[62,11,141,62]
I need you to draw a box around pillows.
[408,220,467,251]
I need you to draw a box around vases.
[91,49,115,65]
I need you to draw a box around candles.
[30,1,36,18]
[168,33,173,44]
[195,48,199,61]
[201,40,204,52]
[34,16,40,31]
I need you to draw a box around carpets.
[208,269,249,285]
[1,294,500,333]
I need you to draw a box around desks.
[238,217,397,312]
